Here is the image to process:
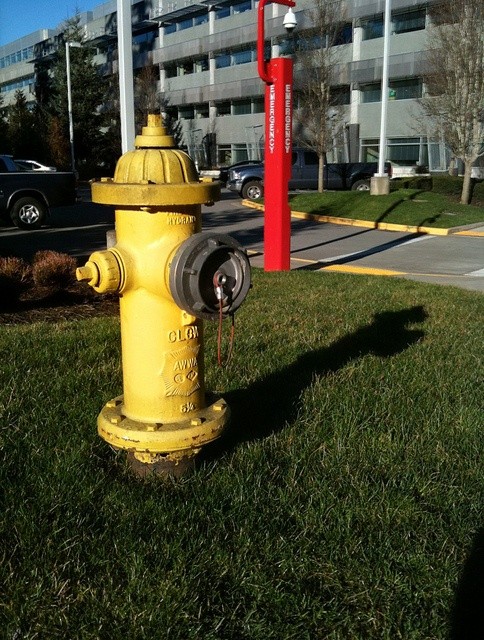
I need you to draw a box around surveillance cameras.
[282,11,297,33]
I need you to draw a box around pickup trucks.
[0,155,73,229]
[226,149,390,200]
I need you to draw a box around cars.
[219,160,261,183]
[13,159,57,172]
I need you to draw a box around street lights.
[64,40,81,168]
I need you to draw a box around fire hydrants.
[73,114,250,479]
